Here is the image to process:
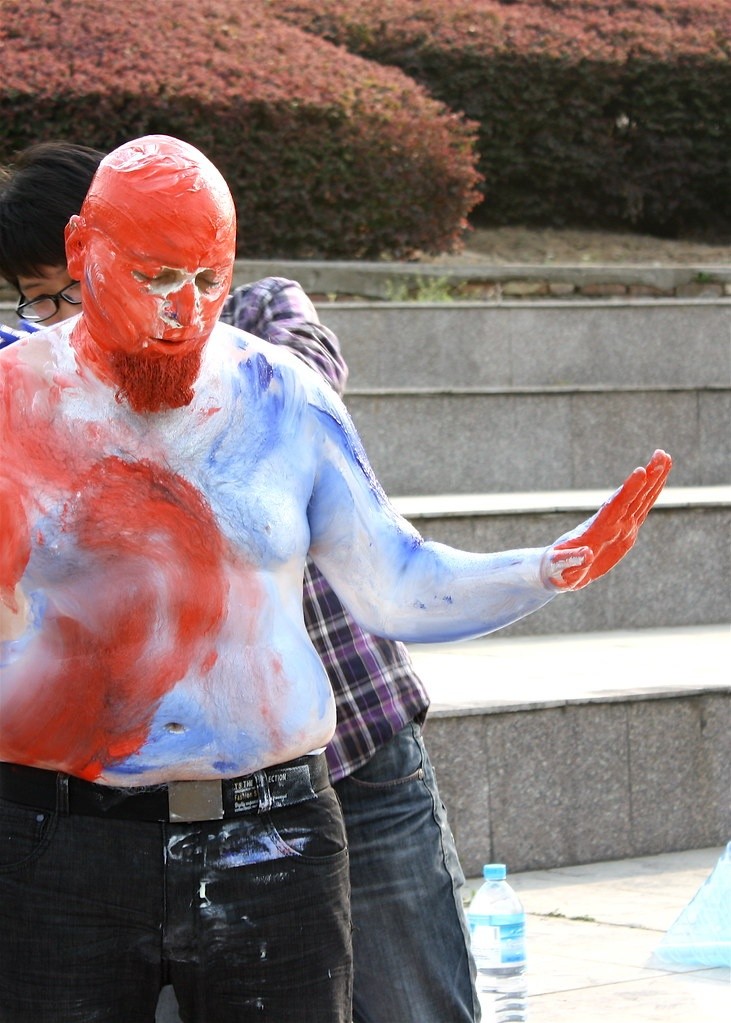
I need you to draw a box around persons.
[2,142,481,1023]
[0,134,675,1023]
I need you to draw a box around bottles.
[464,863,525,1021]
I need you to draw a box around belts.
[2,755,332,825]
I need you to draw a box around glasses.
[15,280,82,324]
